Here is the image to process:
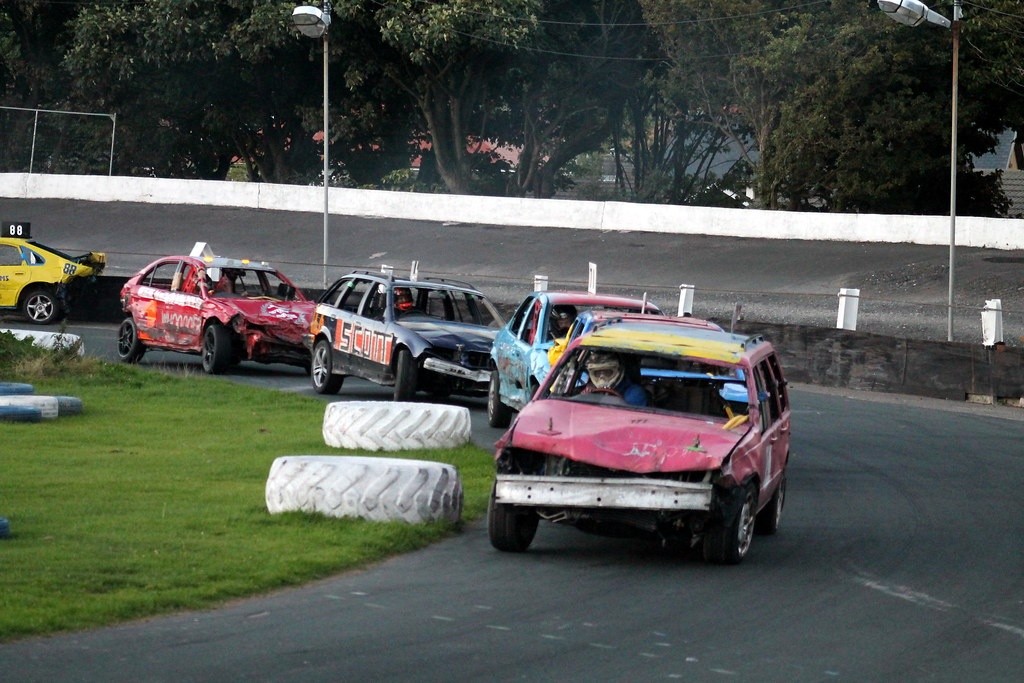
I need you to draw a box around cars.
[118,254,317,374]
[487,312,791,563]
[308,268,506,401]
[489,289,666,427]
[0,236,107,325]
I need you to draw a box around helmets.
[392,287,415,311]
[585,351,625,392]
[550,304,578,339]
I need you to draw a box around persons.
[374,287,420,324]
[547,304,577,342]
[196,270,230,296]
[581,351,647,406]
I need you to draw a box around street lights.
[876,0,958,344]
[293,5,330,290]
